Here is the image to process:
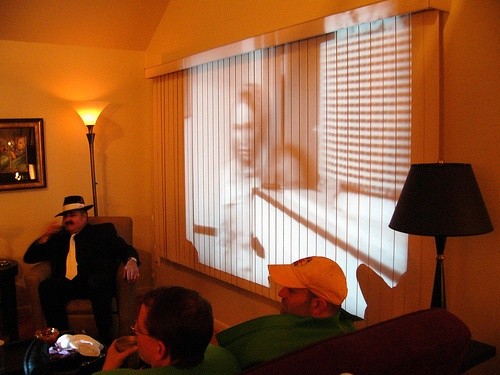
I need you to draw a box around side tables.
[0,259,20,342]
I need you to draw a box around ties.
[66,233,78,281]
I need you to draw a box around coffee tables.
[23,329,130,375]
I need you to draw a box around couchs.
[23,217,134,338]
[239,309,500,375]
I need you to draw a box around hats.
[54,195,93,218]
[268,256,348,306]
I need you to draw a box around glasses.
[131,320,150,336]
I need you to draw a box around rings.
[137,273,140,276]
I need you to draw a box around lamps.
[389,161,494,310]
[70,100,110,217]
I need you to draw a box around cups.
[115,335,138,370]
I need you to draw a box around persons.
[23,194,141,344]
[211,255,353,368]
[213,85,268,290]
[251,133,337,260]
[91,286,243,375]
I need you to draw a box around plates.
[56,334,72,349]
[0,261,10,266]
[68,334,102,356]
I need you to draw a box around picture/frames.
[0,118,47,190]
